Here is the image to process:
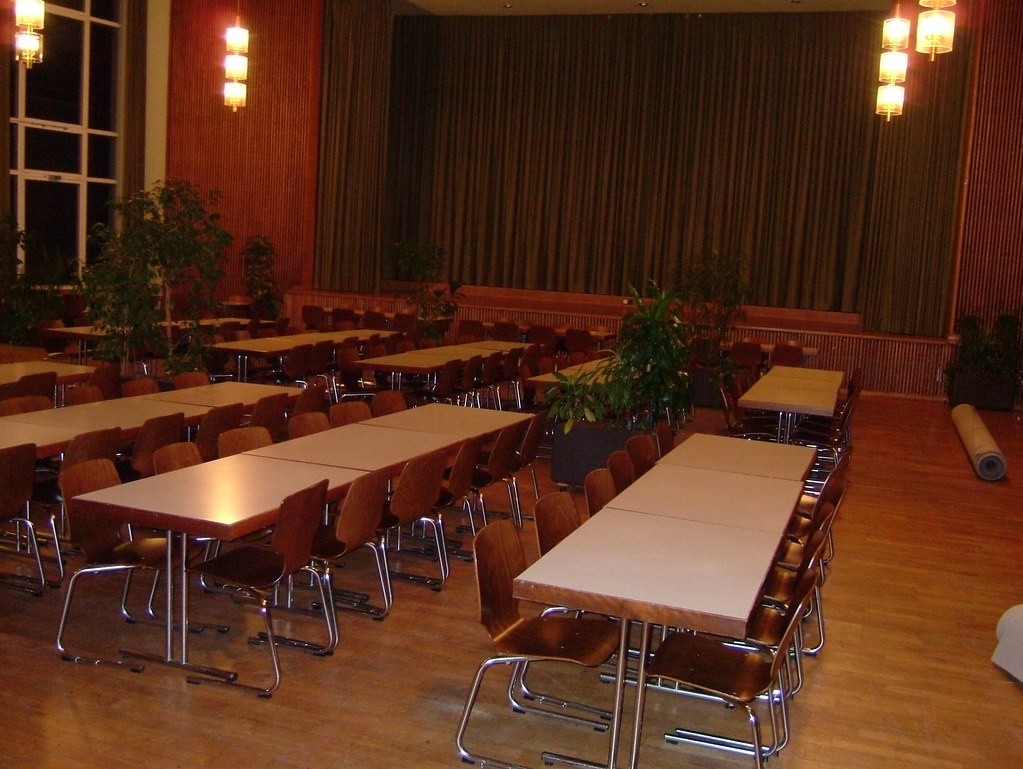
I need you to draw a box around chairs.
[0,293,863,769]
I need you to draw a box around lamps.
[224,0,249,113]
[915,0,956,62]
[15,0,44,69]
[875,0,911,121]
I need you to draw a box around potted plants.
[942,311,1023,412]
[389,238,466,348]
[0,205,71,347]
[241,231,277,320]
[540,283,695,493]
[72,174,236,391]
[684,246,756,407]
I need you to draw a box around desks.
[406,346,508,362]
[457,322,616,350]
[322,307,453,331]
[514,508,783,769]
[655,431,820,481]
[603,464,806,536]
[457,341,545,352]
[722,341,820,369]
[767,366,844,383]
[135,381,306,416]
[0,359,97,409]
[336,329,407,340]
[358,402,534,440]
[353,353,462,405]
[568,359,644,374]
[56,453,371,681]
[204,338,296,382]
[222,301,251,316]
[738,384,837,443]
[43,317,278,366]
[0,417,76,545]
[742,376,839,427]
[270,333,369,344]
[242,424,468,603]
[0,395,214,542]
[526,369,621,386]
